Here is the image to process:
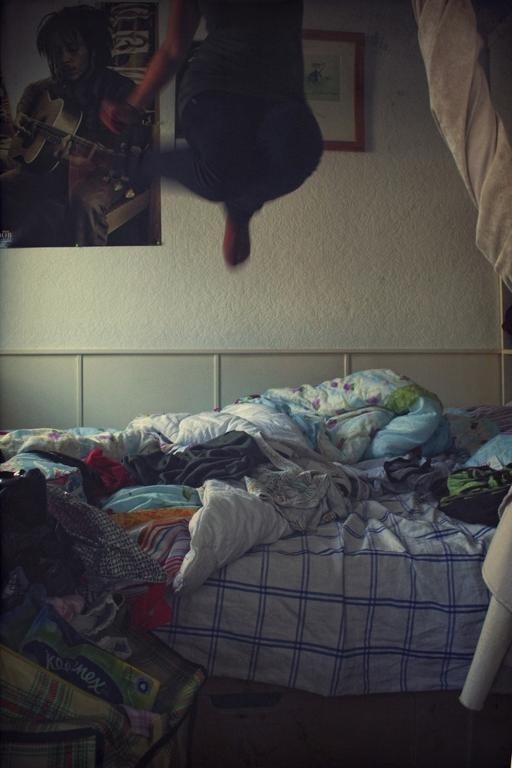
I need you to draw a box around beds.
[2,405,511,697]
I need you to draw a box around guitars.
[7,85,128,179]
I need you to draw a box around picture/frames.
[298,29,365,153]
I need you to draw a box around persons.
[0,3,139,245]
[101,0,325,269]
